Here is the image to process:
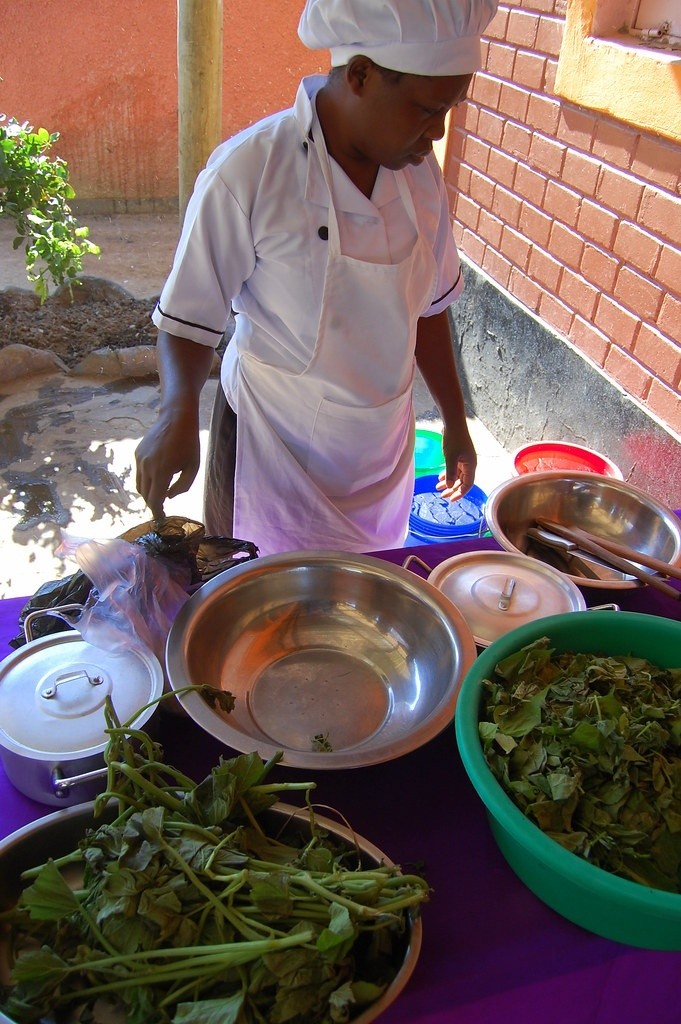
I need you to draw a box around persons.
[132,0,501,571]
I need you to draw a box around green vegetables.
[0,686,431,1024]
[480,636,681,896]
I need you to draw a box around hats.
[297,0,499,76]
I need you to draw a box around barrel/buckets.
[414,429,446,478]
[510,441,624,482]
[403,474,489,547]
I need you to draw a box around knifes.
[525,527,671,583]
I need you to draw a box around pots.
[0,604,164,806]
[402,550,620,650]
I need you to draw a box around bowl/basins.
[454,610,681,951]
[163,549,478,770]
[484,470,681,590]
[0,794,424,1024]
